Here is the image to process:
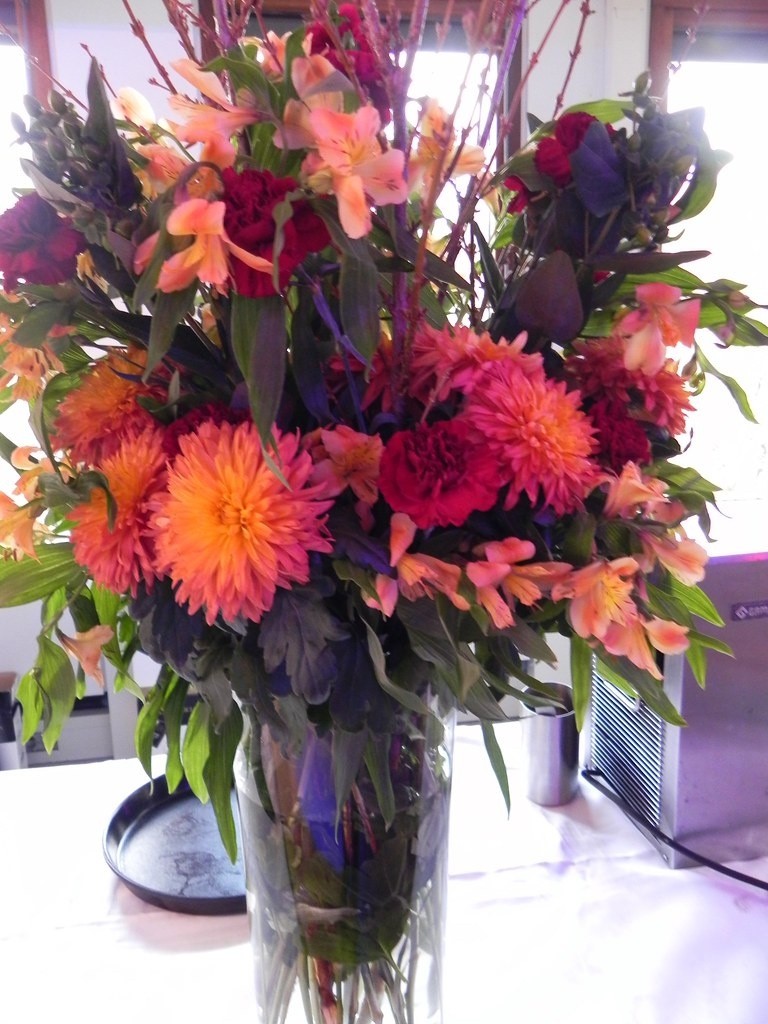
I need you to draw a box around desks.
[0,713,768,1024]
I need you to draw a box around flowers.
[0,0,768,1024]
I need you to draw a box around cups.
[521,681,579,806]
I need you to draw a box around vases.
[233,687,458,1024]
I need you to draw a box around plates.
[102,773,247,916]
